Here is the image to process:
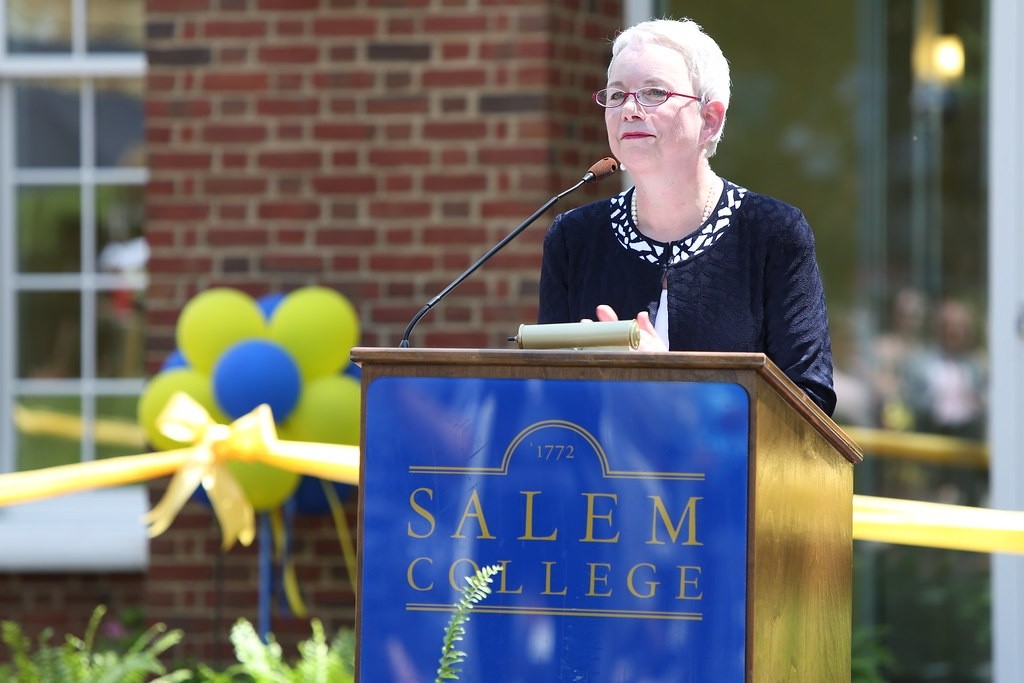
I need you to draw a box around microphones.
[400,156,619,350]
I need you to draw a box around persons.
[535,18,790,417]
[825,287,989,507]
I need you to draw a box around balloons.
[132,277,362,519]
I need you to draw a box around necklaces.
[631,174,716,228]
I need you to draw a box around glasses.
[592,86,707,108]
